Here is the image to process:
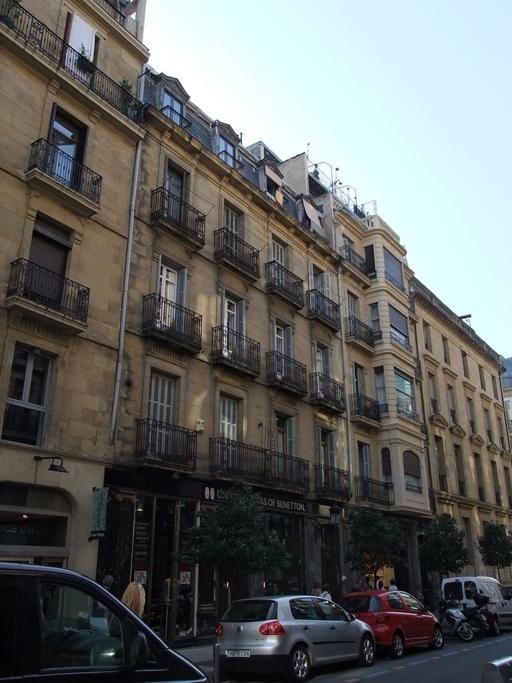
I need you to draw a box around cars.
[0,558,215,682]
[209,593,378,679]
[341,587,446,659]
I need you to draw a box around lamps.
[32,452,71,477]
[328,504,342,529]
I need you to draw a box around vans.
[439,575,511,634]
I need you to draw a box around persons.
[84,570,416,633]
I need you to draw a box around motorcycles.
[461,588,490,636]
[434,592,474,642]
